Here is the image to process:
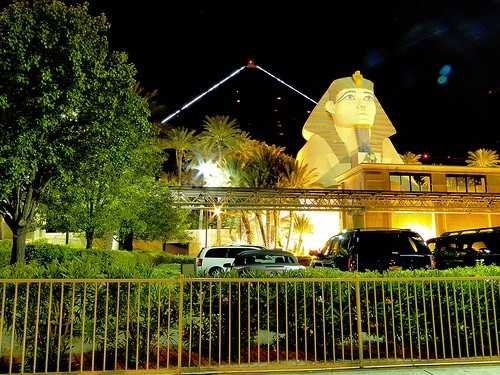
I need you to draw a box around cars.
[224,250,306,277]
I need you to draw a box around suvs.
[427,228,500,270]
[195,245,267,276]
[312,228,431,272]
